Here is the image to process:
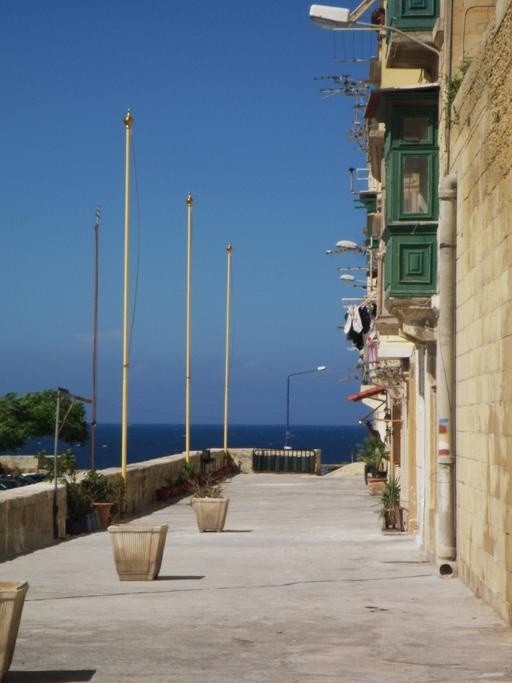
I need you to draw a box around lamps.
[334,236,373,284]
[308,4,443,61]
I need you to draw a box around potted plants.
[355,434,407,532]
[84,472,122,532]
[185,474,232,533]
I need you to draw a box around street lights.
[285,365,325,446]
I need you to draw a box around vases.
[108,522,167,580]
[158,484,182,499]
[1,580,29,678]
